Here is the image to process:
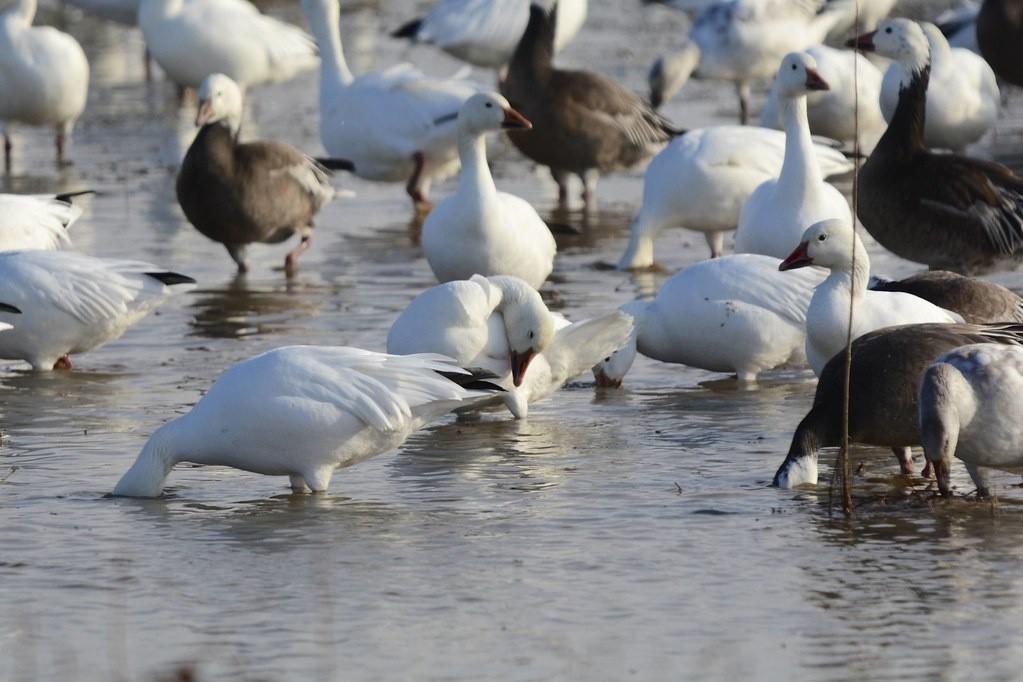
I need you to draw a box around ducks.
[0,2,1023,498]
